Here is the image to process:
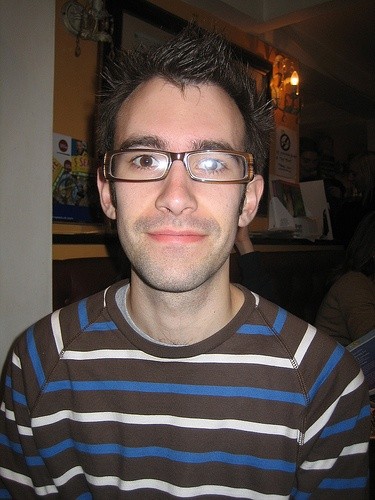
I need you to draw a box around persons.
[280,184,295,208]
[56,139,85,204]
[0,19,374,500]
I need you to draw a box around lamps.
[280,70,299,91]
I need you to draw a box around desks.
[52,221,343,327]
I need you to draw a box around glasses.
[102,149,255,187]
